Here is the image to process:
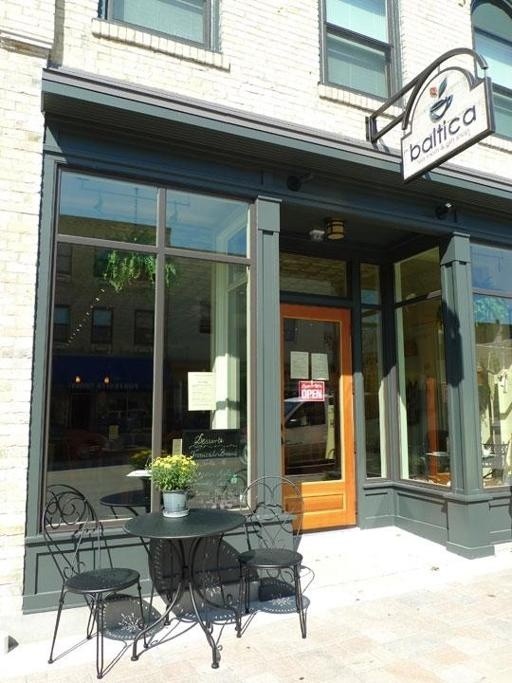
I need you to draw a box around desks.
[100,491,194,516]
[123,509,243,671]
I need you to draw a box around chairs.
[46,484,96,522]
[43,494,148,677]
[239,475,306,640]
[216,468,250,510]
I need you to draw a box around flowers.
[147,455,202,490]
[130,449,166,479]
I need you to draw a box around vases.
[142,480,151,491]
[162,488,187,516]
